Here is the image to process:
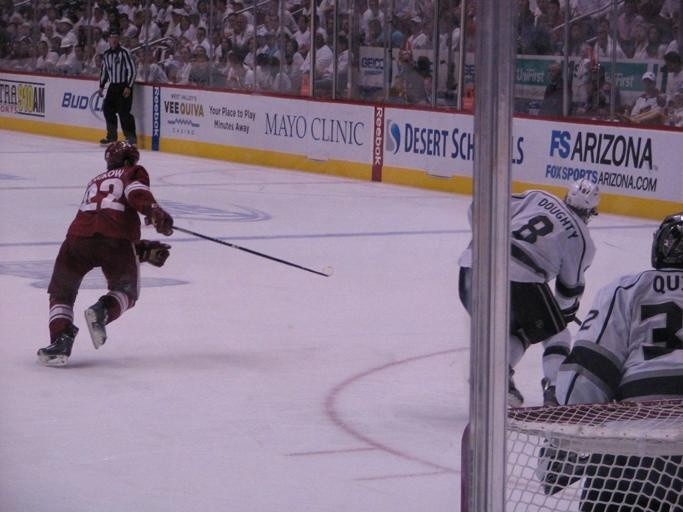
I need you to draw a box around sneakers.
[100,134,137,145]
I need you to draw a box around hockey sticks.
[172,226,333,278]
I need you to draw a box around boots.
[37,322,80,360]
[85,301,110,346]
[506,365,559,409]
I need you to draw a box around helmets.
[103,140,142,164]
[649,210,683,270]
[566,179,599,210]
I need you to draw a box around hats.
[642,70,657,82]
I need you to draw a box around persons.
[453,176,601,408]
[32,140,176,362]
[95,28,140,150]
[536,204,683,510]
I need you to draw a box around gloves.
[150,203,178,238]
[140,240,174,268]
